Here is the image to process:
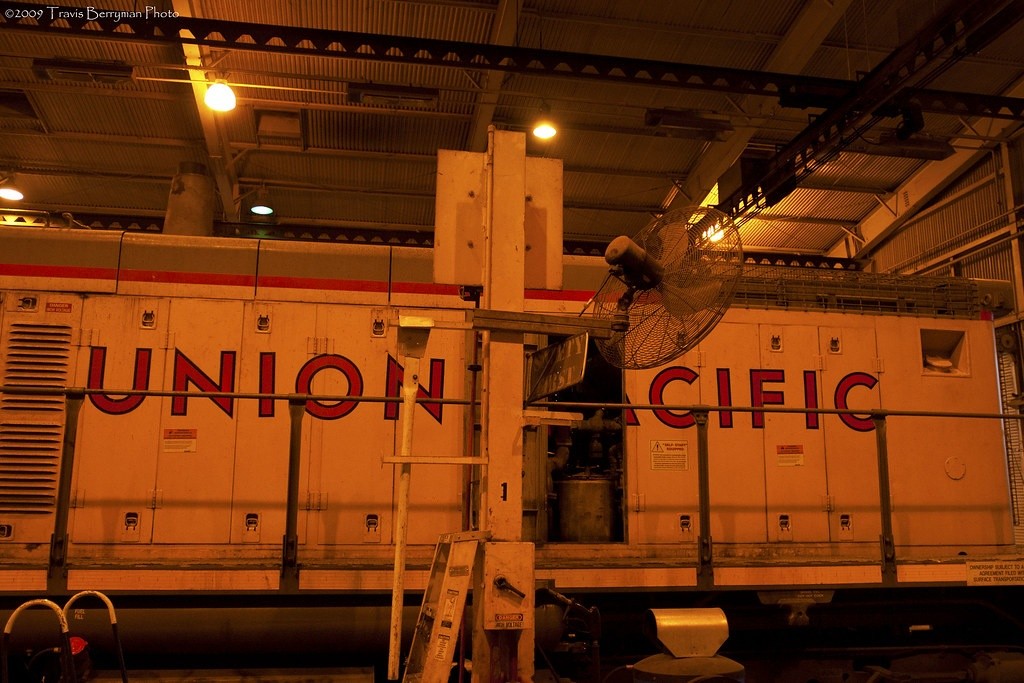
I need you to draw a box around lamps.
[205,71,235,112]
[0,174,24,201]
[530,103,558,140]
[815,143,840,162]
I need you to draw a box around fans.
[593,205,745,370]
